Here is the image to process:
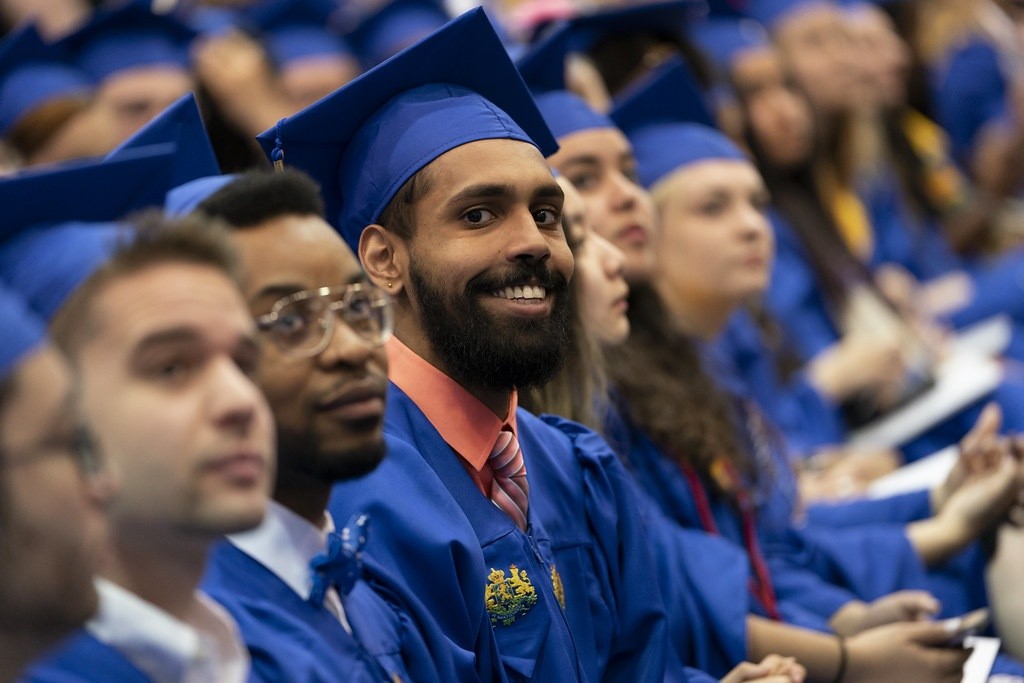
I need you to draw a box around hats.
[256,5,559,255]
[684,16,767,79]
[100,91,243,223]
[507,21,615,139]
[1,0,450,133]
[0,275,46,375]
[600,58,748,188]
[0,143,176,364]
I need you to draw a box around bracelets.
[836,635,848,683]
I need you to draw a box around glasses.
[255,283,393,360]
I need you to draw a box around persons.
[262,7,812,683]
[517,0,1024,683]
[106,93,521,683]
[0,0,508,172]
[0,287,119,683]
[0,143,377,683]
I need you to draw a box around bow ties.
[309,511,376,614]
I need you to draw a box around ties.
[488,429,532,536]
